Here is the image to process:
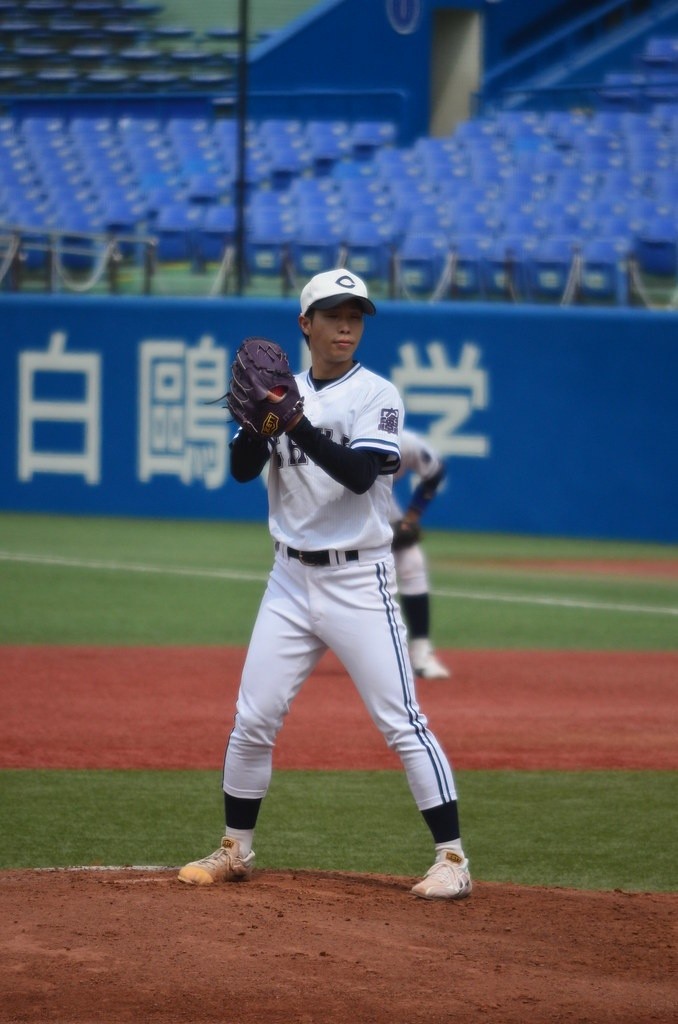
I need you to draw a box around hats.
[300,269,375,317]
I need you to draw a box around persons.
[177,268,473,901]
[390,431,451,678]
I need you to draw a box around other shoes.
[409,648,449,679]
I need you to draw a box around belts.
[276,541,359,566]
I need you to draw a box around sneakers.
[176,835,256,886]
[409,850,472,900]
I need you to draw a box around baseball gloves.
[226,337,303,439]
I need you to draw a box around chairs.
[0,0,678,311]
